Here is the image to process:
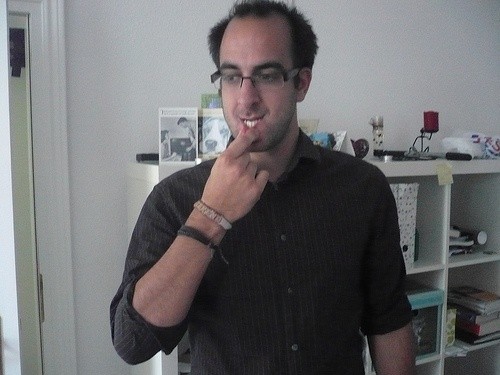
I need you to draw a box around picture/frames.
[197,107,232,162]
[158,107,198,163]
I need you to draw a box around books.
[449,229,475,255]
[447,286,499,346]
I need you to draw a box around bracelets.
[190,198,237,228]
[177,224,229,264]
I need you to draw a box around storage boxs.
[406,280,444,361]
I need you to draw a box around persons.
[161,117,196,162]
[110,0,416,375]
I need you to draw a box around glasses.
[211,67,299,89]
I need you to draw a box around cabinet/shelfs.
[130,159,500,375]
